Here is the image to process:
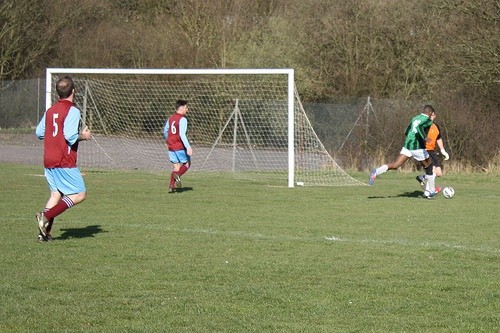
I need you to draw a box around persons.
[369,104,449,199]
[164,100,192,193]
[35,75,92,243]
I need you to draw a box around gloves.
[440,148,449,161]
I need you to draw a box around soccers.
[443,186,455,199]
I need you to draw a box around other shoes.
[416,175,426,185]
[169,188,182,192]
[370,168,377,185]
[425,190,438,197]
[172,172,181,188]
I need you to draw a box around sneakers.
[38,233,52,241]
[36,212,49,237]
